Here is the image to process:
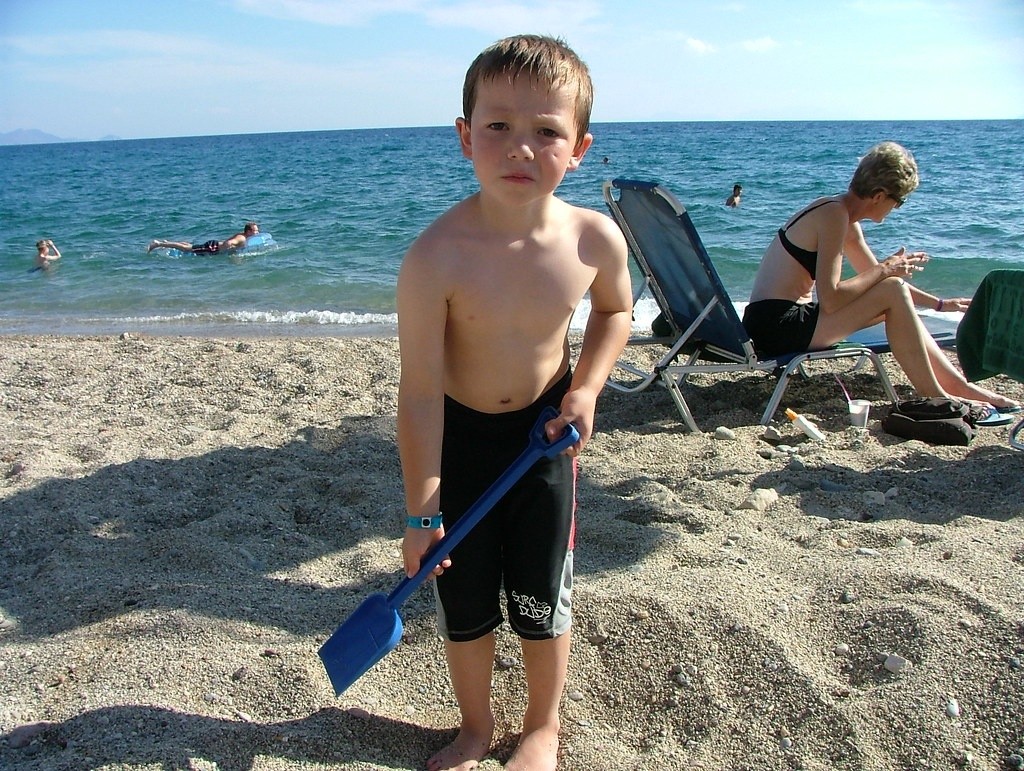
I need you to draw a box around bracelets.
[405,512,443,529]
[936,300,943,311]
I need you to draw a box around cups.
[848,399,870,427]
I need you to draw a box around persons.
[395,34,633,771]
[148,223,259,256]
[726,185,742,206]
[36,240,61,271]
[741,142,1021,420]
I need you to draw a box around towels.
[956,268,1023,384]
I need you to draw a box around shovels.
[318,404,581,699]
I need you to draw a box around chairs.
[957,269,1024,452]
[602,179,956,433]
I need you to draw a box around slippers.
[996,406,1021,414]
[976,408,1014,426]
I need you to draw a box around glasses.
[872,186,907,209]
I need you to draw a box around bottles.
[785,409,825,441]
